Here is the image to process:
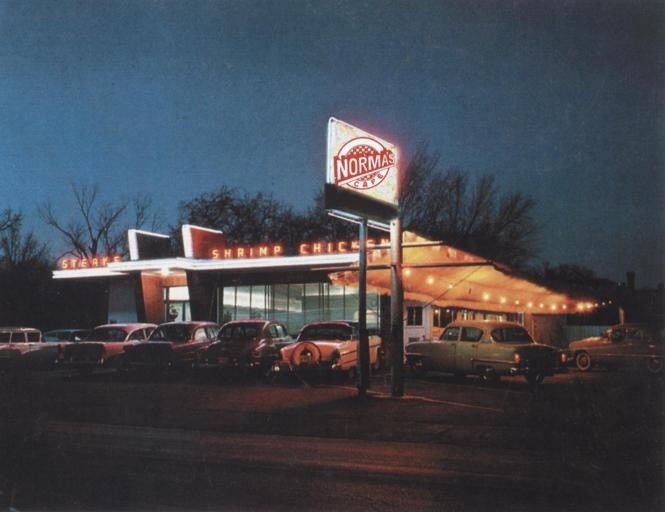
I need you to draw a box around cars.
[122,320,221,373]
[405,319,568,387]
[566,322,664,375]
[272,319,385,378]
[190,319,292,372]
[0,326,91,369]
[64,321,160,375]
